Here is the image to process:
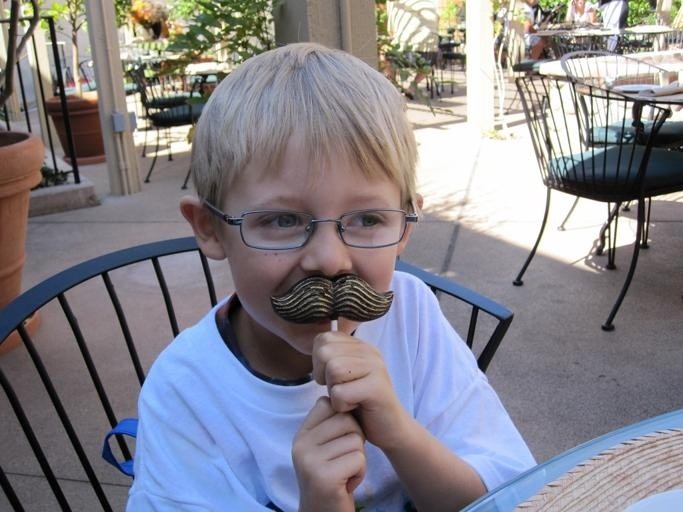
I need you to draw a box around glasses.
[200,198,418,251]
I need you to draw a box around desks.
[459,409,682,512]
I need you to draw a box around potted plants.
[0,0,45,355]
[19,0,132,165]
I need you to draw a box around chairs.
[79,59,206,189]
[0,236,512,511]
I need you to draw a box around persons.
[507,1,562,61]
[381,0,440,102]
[122,40,536,512]
[598,0,628,54]
[564,0,597,51]
